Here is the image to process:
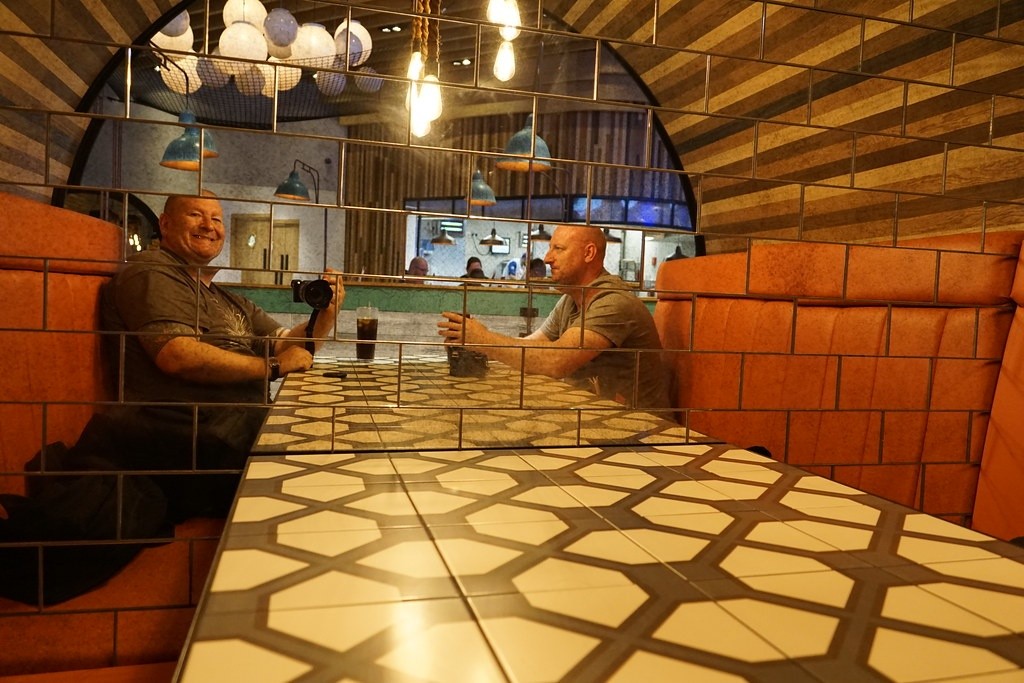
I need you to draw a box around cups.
[356,305,380,359]
[449,311,471,345]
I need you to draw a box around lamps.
[486,0,523,83]
[664,233,690,263]
[480,226,506,246]
[272,158,325,205]
[404,0,444,138]
[178,110,220,162]
[432,227,454,245]
[495,113,575,223]
[529,224,552,241]
[146,39,204,170]
[601,227,622,245]
[464,146,566,223]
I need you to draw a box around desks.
[249,355,726,455]
[161,458,1024,681]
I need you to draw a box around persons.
[401,258,429,285]
[437,221,679,427]
[110,188,345,446]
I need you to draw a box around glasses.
[414,266,429,272]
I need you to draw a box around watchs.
[267,356,281,383]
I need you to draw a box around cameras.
[449,346,487,379]
[291,279,333,312]
